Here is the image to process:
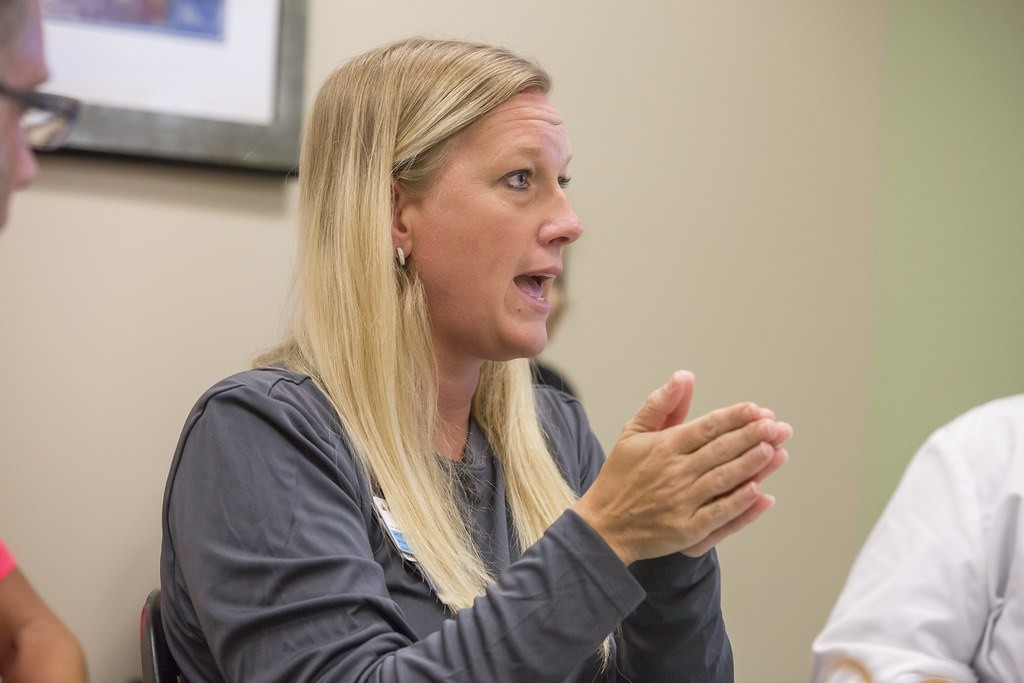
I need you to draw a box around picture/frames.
[24,0,311,177]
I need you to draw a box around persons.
[811,394,1024,683]
[161,38,794,683]
[0,0,92,683]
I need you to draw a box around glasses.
[0,84,81,150]
